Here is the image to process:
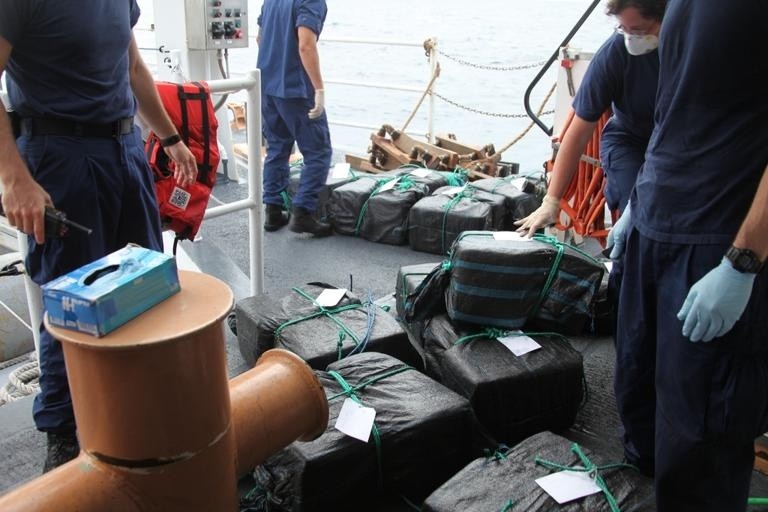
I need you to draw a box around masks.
[623,32,660,56]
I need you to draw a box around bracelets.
[158,133,179,147]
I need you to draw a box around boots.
[42,432,81,475]
[264,203,291,232]
[288,207,333,235]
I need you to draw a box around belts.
[17,117,134,137]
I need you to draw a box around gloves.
[512,194,563,238]
[676,254,758,343]
[606,199,633,259]
[308,89,325,119]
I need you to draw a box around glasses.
[615,21,657,36]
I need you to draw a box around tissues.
[38,242,181,339]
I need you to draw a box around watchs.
[727,244,768,275]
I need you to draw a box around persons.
[512,1,670,348]
[605,1,767,512]
[0,1,200,474]
[256,0,334,236]
